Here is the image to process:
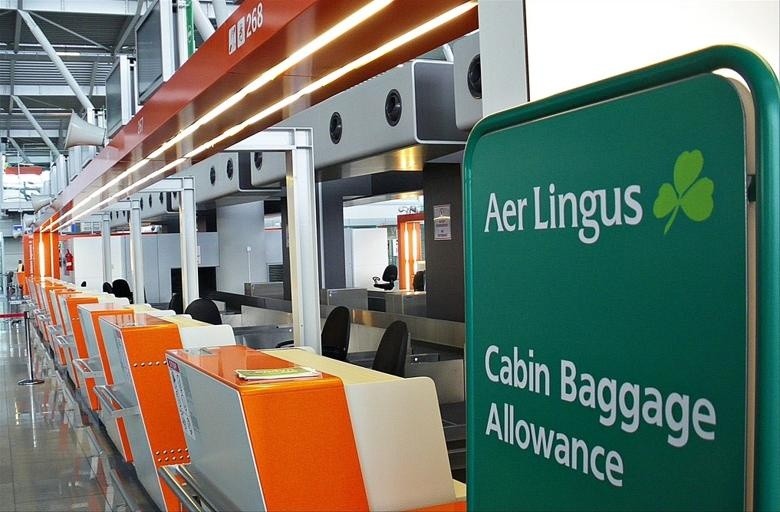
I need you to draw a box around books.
[232,364,321,381]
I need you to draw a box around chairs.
[277,305,410,377]
[168,292,249,325]
[373,263,398,291]
[105,279,135,305]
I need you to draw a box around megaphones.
[31,193,50,214]
[64,108,106,151]
[12,229,22,240]
[23,213,34,227]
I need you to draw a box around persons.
[16,259,24,272]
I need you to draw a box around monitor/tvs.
[80,108,96,169]
[49,166,57,201]
[68,145,81,181]
[134,0,176,106]
[105,54,132,139]
[56,154,67,195]
